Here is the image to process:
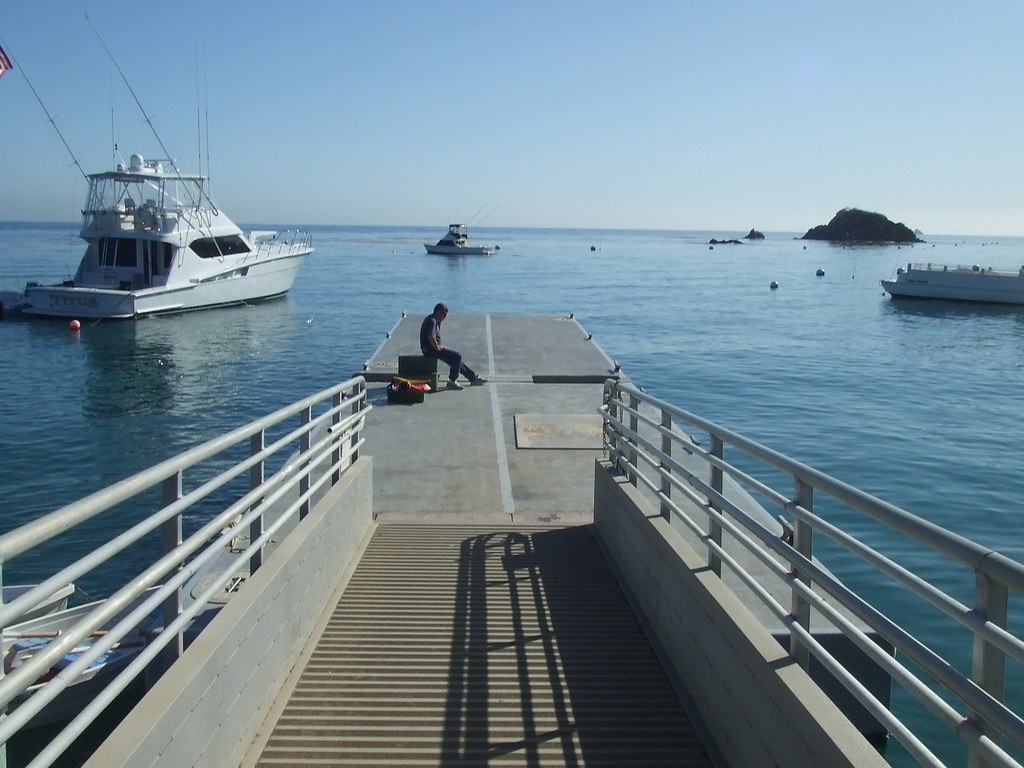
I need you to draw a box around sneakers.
[446,381,464,390]
[471,374,488,386]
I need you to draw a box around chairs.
[147,200,157,213]
[124,198,138,213]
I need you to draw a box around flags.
[0,44,13,80]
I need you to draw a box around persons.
[420,303,488,390]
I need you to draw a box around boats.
[0,582,75,628]
[0,22,315,320]
[879,262,1024,305]
[0,584,168,730]
[423,201,500,255]
